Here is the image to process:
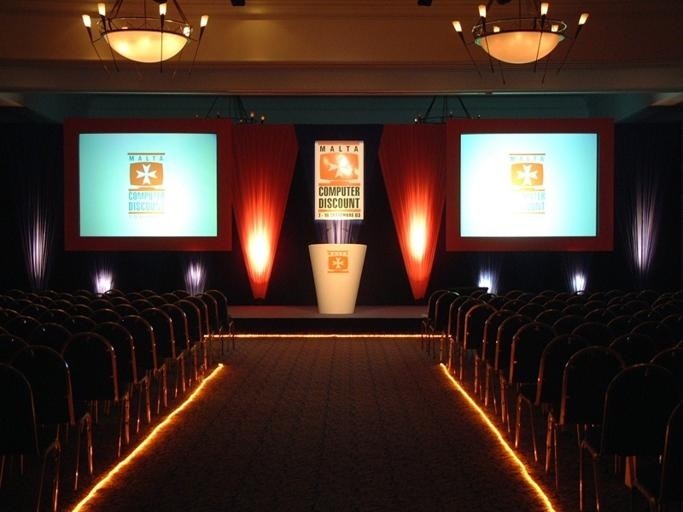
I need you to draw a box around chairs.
[416,283,682,512]
[0,283,237,512]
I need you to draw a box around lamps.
[451,0,593,89]
[81,0,209,76]
